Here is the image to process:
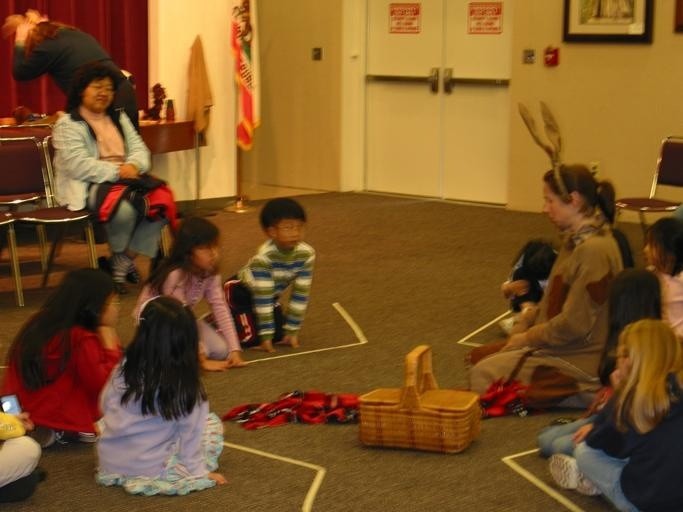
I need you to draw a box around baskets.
[356,343,484,456]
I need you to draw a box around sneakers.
[548,453,604,496]
[96,253,140,296]
[24,424,100,449]
[498,317,514,335]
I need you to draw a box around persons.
[499,215,681,341]
[199,195,318,357]
[125,213,247,374]
[0,7,140,137]
[535,264,663,460]
[466,162,637,417]
[546,317,682,511]
[0,391,47,506]
[45,63,173,297]
[88,295,229,498]
[0,264,125,452]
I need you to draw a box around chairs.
[0,137,100,306]
[0,210,28,306]
[42,132,170,292]
[611,135,682,249]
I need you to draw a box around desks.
[0,111,208,154]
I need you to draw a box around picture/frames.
[672,1,682,33]
[562,1,654,47]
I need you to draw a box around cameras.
[0,393,23,416]
